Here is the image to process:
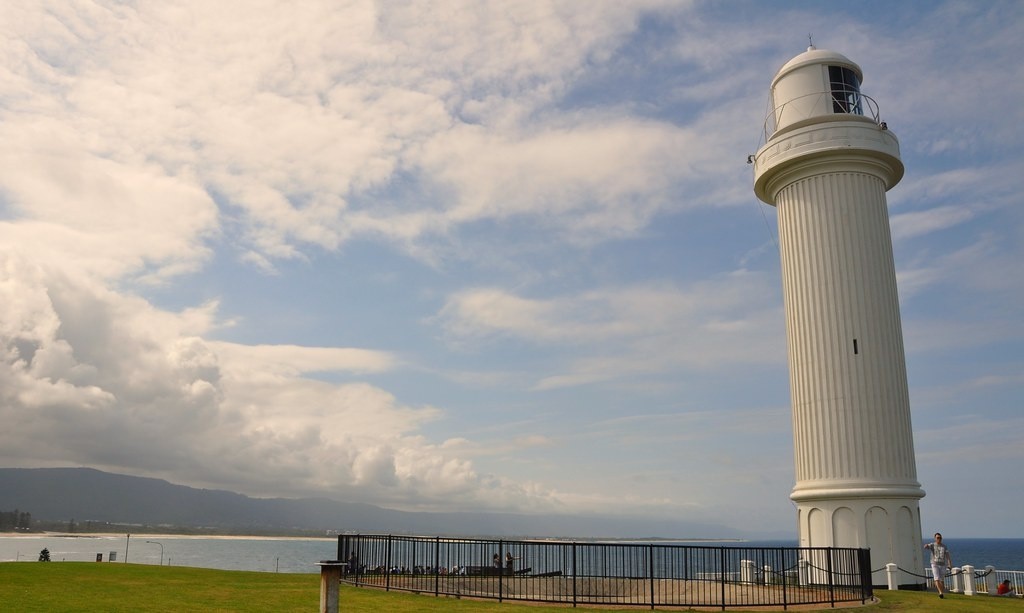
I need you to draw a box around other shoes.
[938,593,944,599]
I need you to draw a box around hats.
[1003,579,1011,584]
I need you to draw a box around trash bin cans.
[109,551,117,561]
[96,553,102,562]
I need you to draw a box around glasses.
[937,537,943,539]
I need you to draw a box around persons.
[349,551,357,575]
[493,552,503,568]
[357,565,465,575]
[924,533,952,600]
[506,552,522,569]
[998,579,1024,599]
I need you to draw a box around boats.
[352,562,563,579]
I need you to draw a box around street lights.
[146,541,163,566]
[125,533,131,562]
